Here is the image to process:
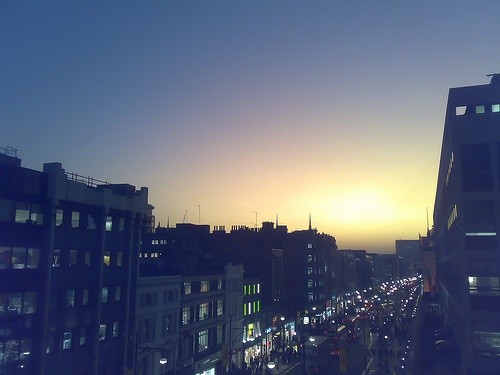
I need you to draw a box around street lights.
[265,328,271,363]
[293,330,306,375]
[281,315,286,352]
[361,307,367,344]
[308,306,316,330]
[332,319,339,356]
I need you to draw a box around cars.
[324,299,394,343]
[330,342,342,356]
